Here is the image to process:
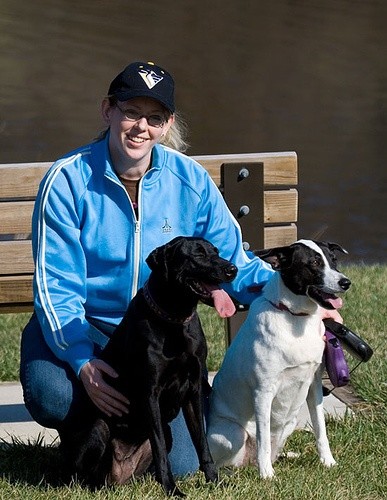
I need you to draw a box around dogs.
[206,239,351,481]
[61,236,238,500]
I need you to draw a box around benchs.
[0,152,297,368]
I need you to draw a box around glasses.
[114,105,164,128]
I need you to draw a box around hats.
[106,62,175,117]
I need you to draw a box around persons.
[21,62,345,482]
